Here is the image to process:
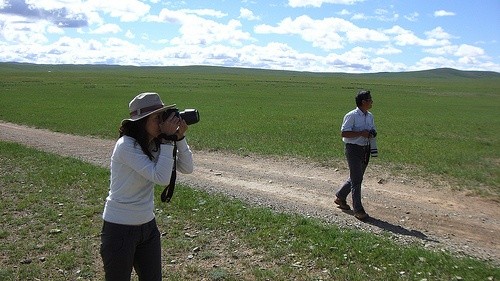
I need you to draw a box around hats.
[121,93,176,126]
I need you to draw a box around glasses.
[366,99,373,101]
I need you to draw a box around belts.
[346,145,367,149]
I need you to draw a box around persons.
[333,91,377,222]
[101,93,194,281]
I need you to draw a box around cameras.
[370,130,379,156]
[164,108,199,126]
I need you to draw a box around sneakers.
[353,208,368,220]
[334,197,350,210]
